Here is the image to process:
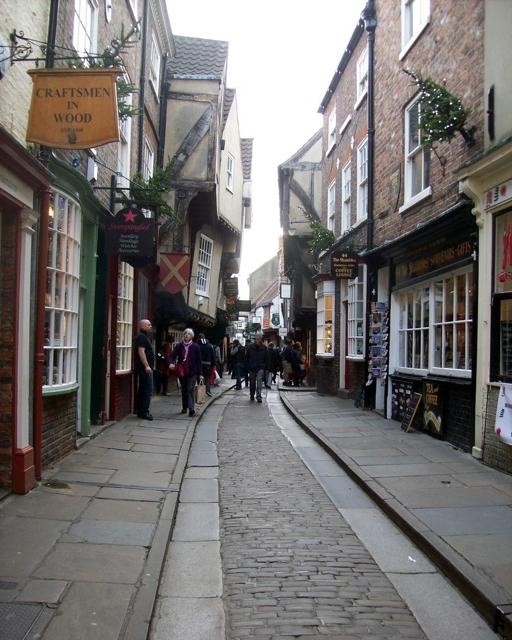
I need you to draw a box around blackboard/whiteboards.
[400,392,423,433]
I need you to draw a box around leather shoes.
[137,414,153,421]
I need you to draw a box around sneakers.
[181,409,195,417]
[250,395,262,403]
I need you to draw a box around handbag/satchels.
[195,378,206,404]
[210,368,221,385]
[174,362,185,377]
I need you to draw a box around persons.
[167,328,205,416]
[244,332,269,403]
[154,334,174,397]
[196,333,310,390]
[197,335,217,398]
[133,318,157,420]
